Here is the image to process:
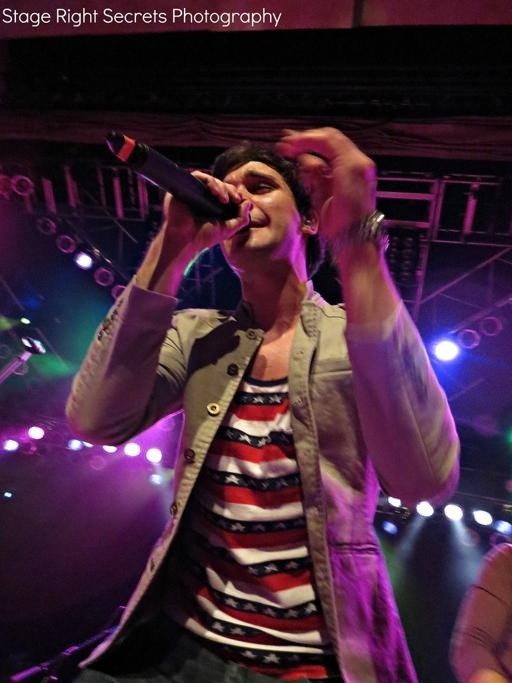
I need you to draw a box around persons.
[64,125,461,683]
[448,542,512,683]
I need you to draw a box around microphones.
[105,128,250,234]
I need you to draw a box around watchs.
[331,210,387,248]
